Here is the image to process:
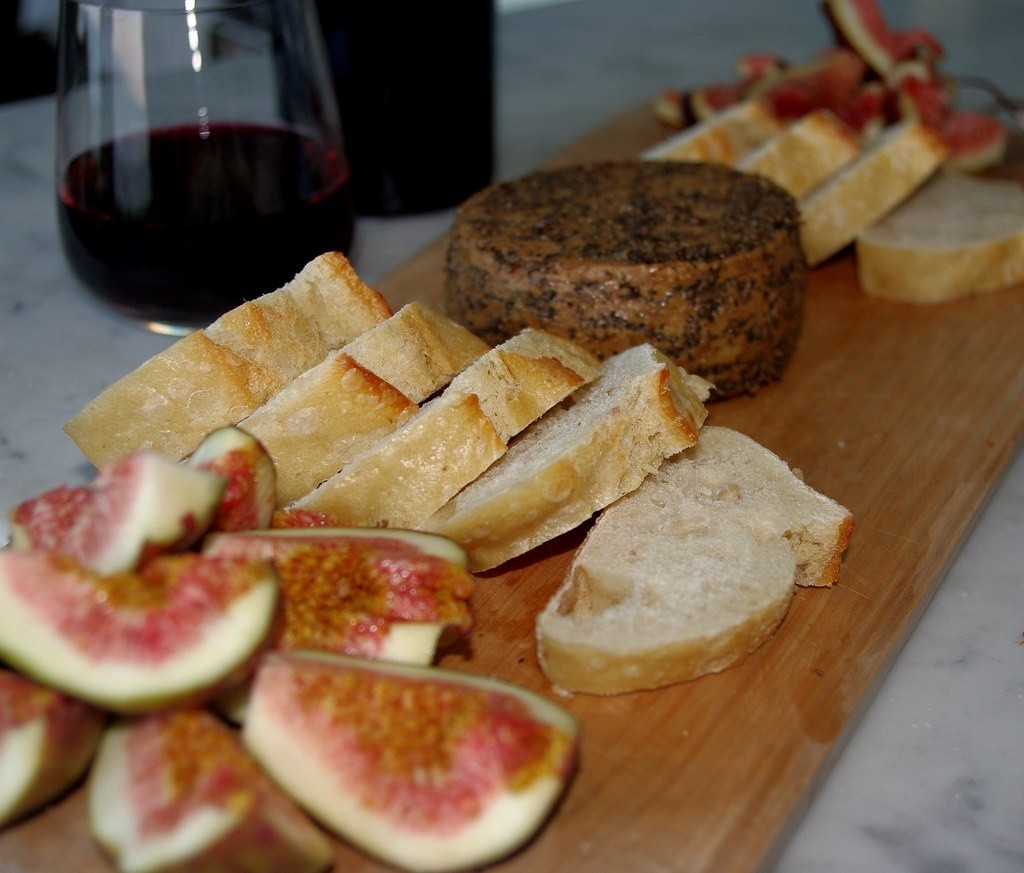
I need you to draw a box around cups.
[48,0,360,335]
[279,0,497,218]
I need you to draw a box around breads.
[635,99,1023,300]
[66,253,854,693]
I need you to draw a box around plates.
[0,98,1021,873]
